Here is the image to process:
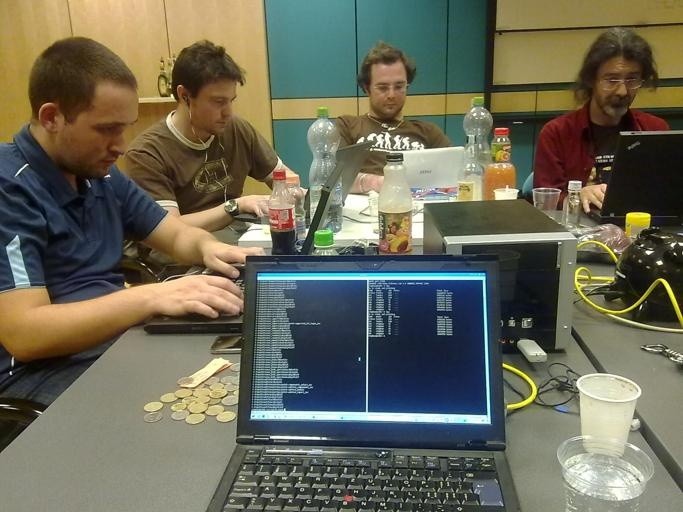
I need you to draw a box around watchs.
[224,199,240,216]
[0,36,266,407]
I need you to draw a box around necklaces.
[367,113,404,131]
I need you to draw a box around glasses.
[598,76,645,91]
[374,82,410,92]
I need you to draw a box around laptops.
[144,161,344,335]
[233,140,375,225]
[583,131,683,225]
[388,146,465,190]
[206,254,522,511]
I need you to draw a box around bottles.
[457,96,516,201]
[156,53,176,98]
[563,181,582,228]
[378,153,413,256]
[269,107,344,255]
[625,212,651,239]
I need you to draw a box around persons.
[533,28,671,214]
[124,40,304,283]
[335,43,455,194]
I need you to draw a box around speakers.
[421,197,578,354]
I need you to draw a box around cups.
[557,436,654,512]
[532,187,562,220]
[576,373,642,457]
[493,188,519,200]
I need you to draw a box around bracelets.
[359,174,369,193]
